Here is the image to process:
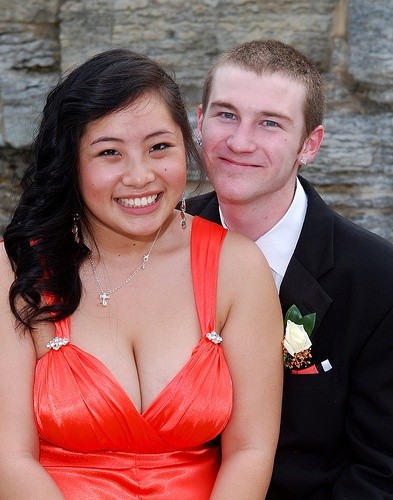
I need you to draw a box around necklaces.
[76,222,164,306]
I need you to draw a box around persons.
[171,40,393,500]
[0,48,284,500]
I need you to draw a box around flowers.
[282,306,317,370]
